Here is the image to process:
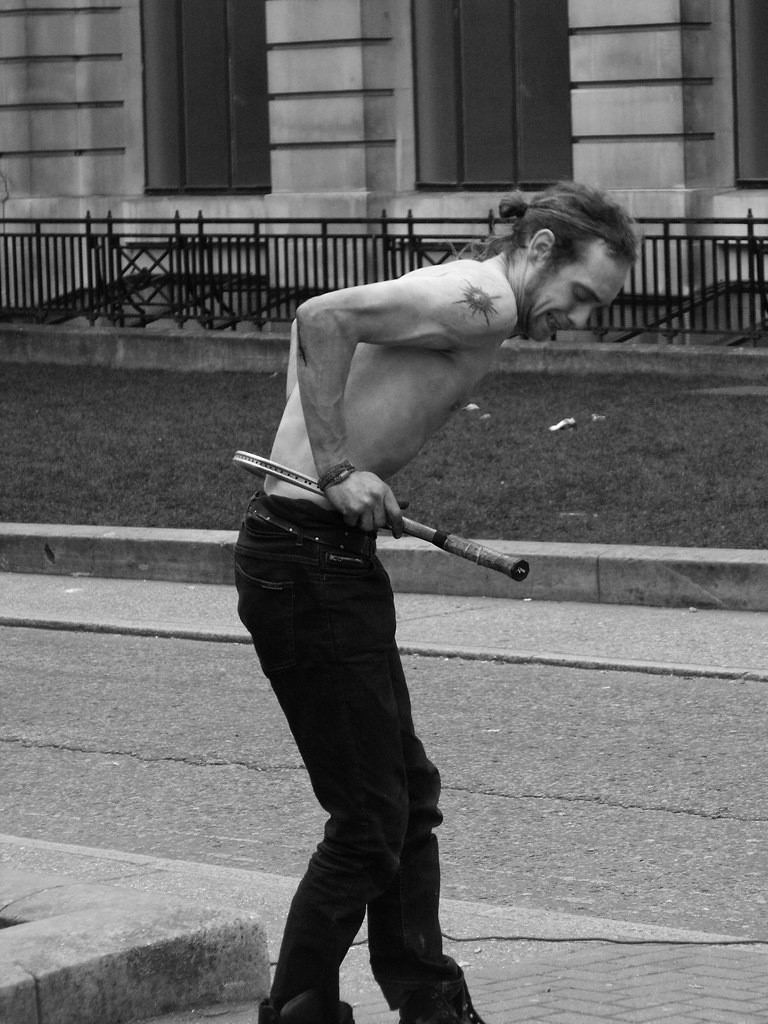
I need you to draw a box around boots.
[258,990,356,1024]
[395,979,486,1024]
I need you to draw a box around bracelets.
[317,459,356,492]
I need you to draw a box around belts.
[248,496,374,553]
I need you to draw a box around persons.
[234,180,637,1024]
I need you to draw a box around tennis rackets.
[232,449,532,581]
